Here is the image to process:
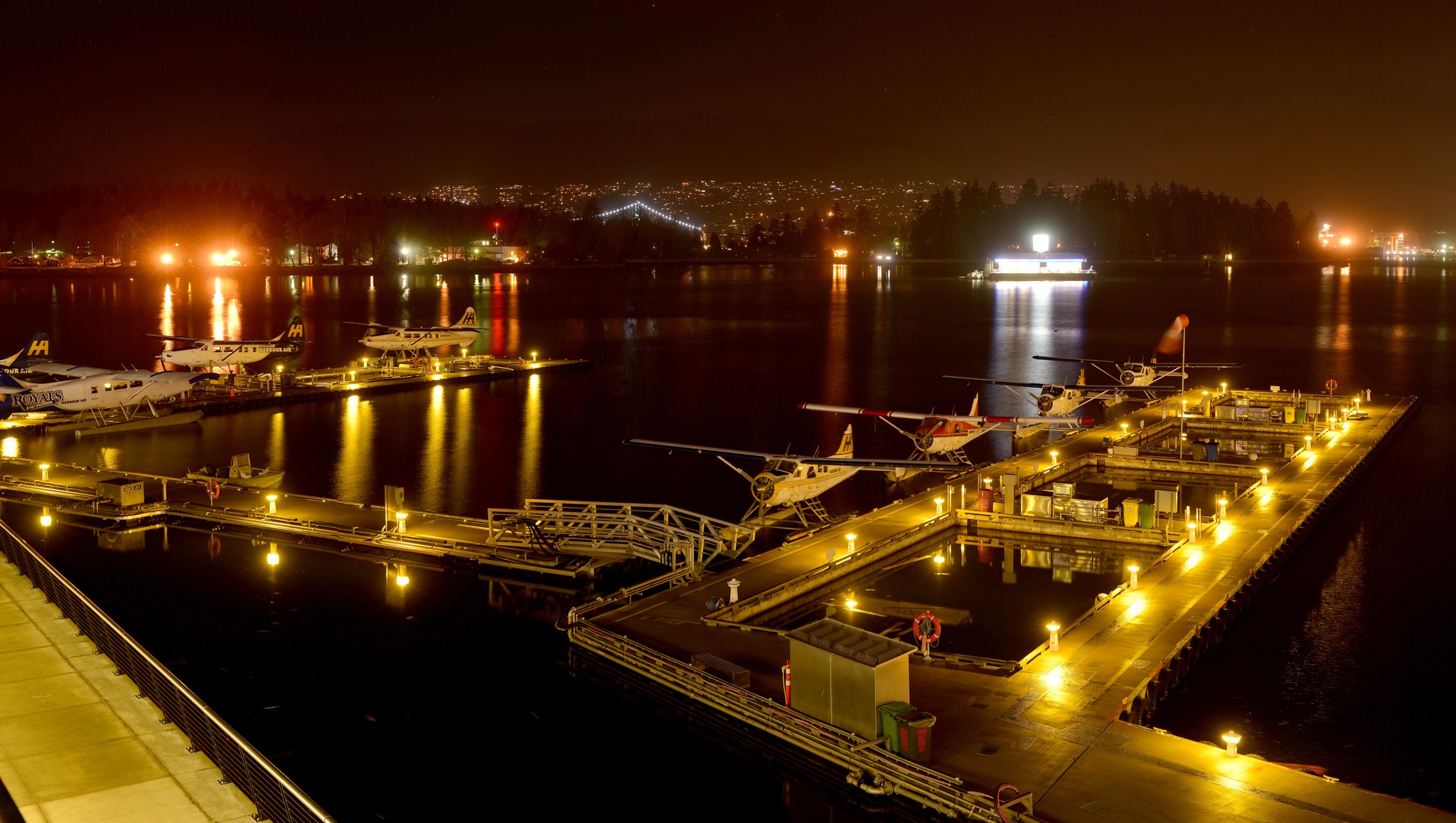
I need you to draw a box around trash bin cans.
[877,701,917,757]
[893,709,936,768]
[1139,500,1156,529]
[1121,497,1144,527]
[1295,407,1306,424]
[1189,441,1206,461]
[1284,406,1296,424]
[1204,442,1220,462]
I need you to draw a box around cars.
[51,249,56,251]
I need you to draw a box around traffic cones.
[430,366,435,376]
[230,386,236,396]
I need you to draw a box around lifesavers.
[913,613,941,643]
[207,535,221,556]
[1325,379,1337,391]
[206,479,219,498]
[413,356,420,365]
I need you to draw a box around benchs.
[691,651,750,689]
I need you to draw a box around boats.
[964,273,982,279]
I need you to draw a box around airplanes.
[142,316,317,378]
[796,392,1095,480]
[620,424,985,542]
[1031,349,1248,409]
[0,354,225,435]
[942,368,1189,438]
[341,306,492,365]
